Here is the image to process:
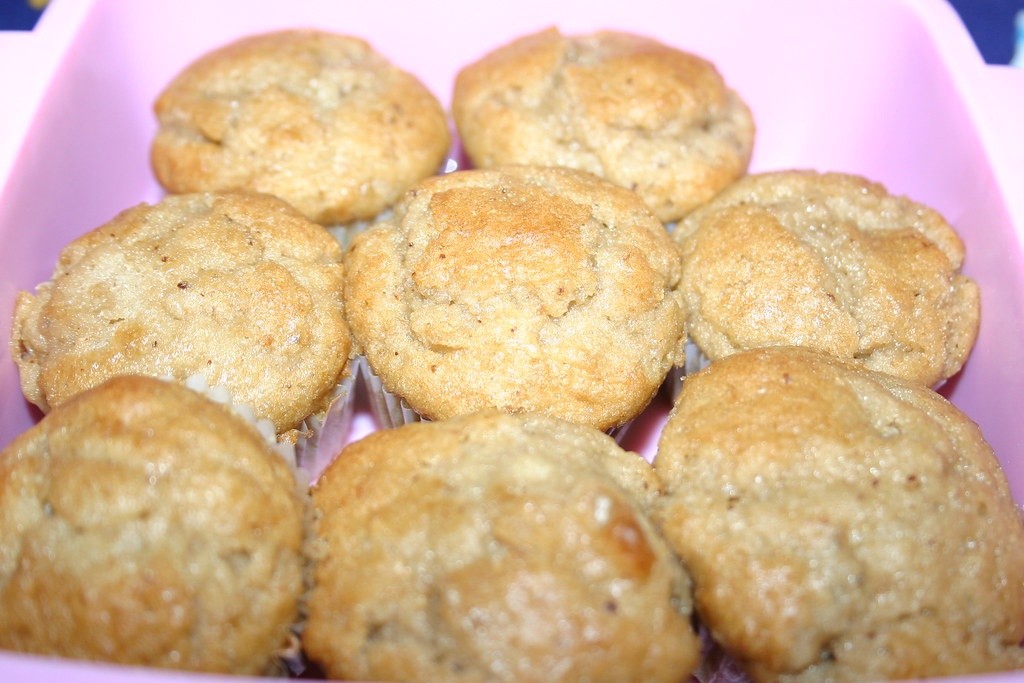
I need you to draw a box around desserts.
[0,27,1024,683]
